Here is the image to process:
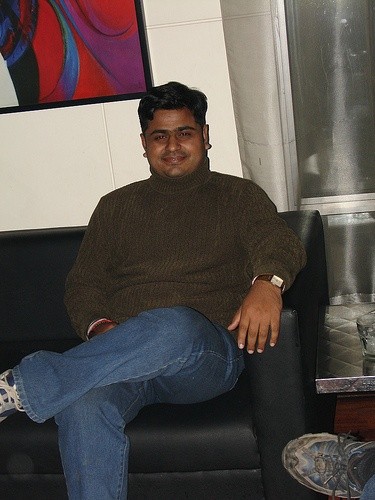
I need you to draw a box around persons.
[281,432,375,500]
[0,82,307,500]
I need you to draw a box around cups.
[356,314,375,359]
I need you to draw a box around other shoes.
[277,432,375,500]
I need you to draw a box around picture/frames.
[0,0,155,114]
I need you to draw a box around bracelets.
[85,316,117,340]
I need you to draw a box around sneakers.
[0,369,21,424]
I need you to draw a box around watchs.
[256,274,285,288]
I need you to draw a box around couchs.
[1,210,336,500]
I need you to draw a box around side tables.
[316,302,375,443]
